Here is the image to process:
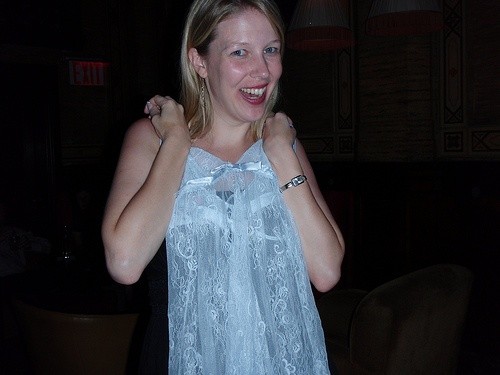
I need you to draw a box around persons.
[99,0,346,375]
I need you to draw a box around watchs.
[280,174,307,192]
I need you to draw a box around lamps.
[365,0,444,37]
[285,0,354,53]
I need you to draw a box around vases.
[12,281,153,375]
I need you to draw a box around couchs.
[317,264,472,375]
